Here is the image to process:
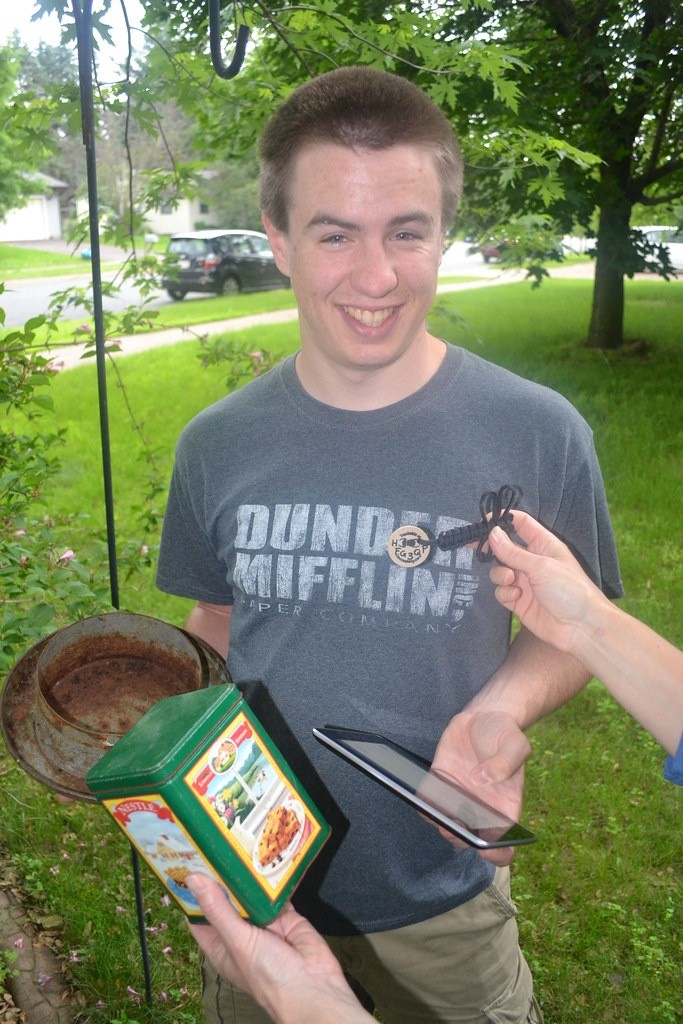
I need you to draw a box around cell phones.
[312,727,539,850]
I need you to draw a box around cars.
[481,225,683,279]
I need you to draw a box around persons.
[184,509,683,1023]
[155,66,626,1023]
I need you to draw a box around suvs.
[164,230,290,299]
